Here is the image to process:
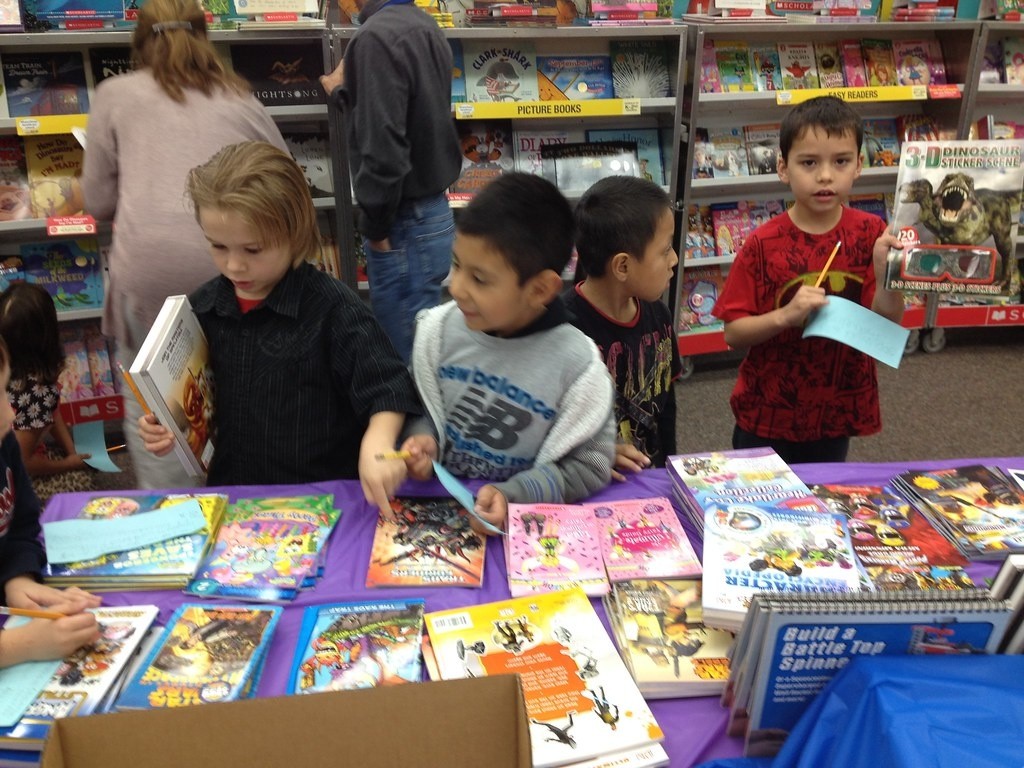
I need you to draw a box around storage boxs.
[38,671,533,768]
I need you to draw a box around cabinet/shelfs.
[0,24,1024,431]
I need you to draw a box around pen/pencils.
[376,452,412,460]
[107,445,127,452]
[117,362,152,415]
[815,241,841,287]
[0,606,62,620]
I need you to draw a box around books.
[0,0,1024,403]
[0,445,1024,768]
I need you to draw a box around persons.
[319,0,464,368]
[0,332,101,672]
[396,171,617,537]
[0,282,94,504]
[562,176,682,481]
[138,140,425,524]
[710,96,905,462]
[79,0,294,488]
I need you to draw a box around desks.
[0,456,1024,768]
